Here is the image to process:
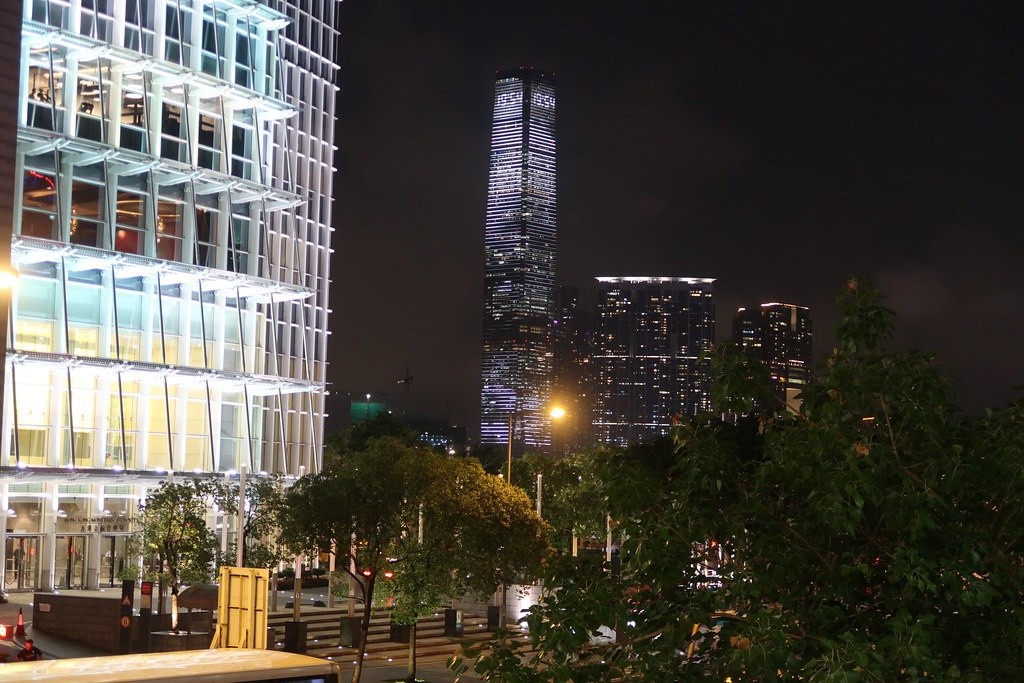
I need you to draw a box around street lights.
[499,407,565,637]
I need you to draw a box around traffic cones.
[13,607,29,636]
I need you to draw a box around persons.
[15,639,43,662]
[13,543,26,579]
